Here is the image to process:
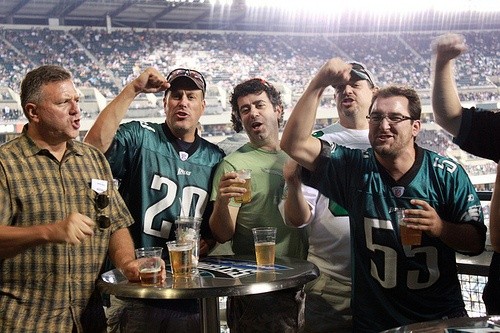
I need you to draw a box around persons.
[80,67,226,333]
[416,145,496,192]
[0,24,500,144]
[279,57,486,333]
[275,59,380,332]
[431,34,500,316]
[479,200,491,245]
[0,67,168,333]
[456,273,489,318]
[208,78,312,333]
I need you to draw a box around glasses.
[165,68,207,87]
[94,191,111,229]
[366,112,420,126]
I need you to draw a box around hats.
[331,60,375,88]
[167,69,207,92]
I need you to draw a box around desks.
[101,256,321,333]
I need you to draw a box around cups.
[134,247,163,287]
[397,210,422,245]
[174,215,201,268]
[229,169,252,203]
[166,240,196,279]
[251,227,277,267]
